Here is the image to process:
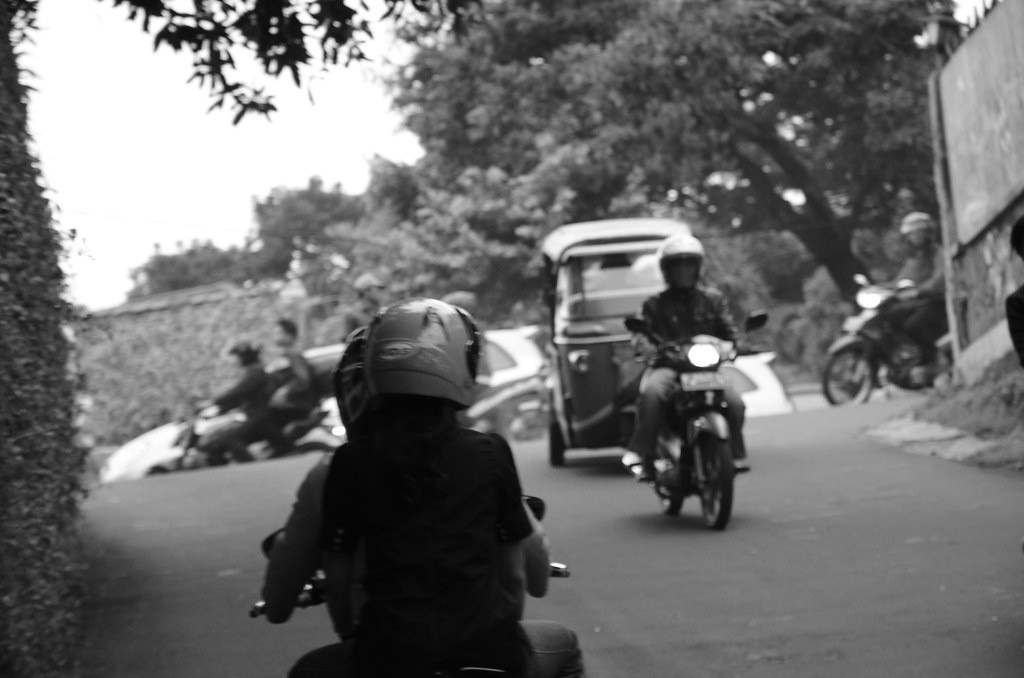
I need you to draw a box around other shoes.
[641,460,653,479]
[734,454,746,471]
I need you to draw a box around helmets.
[333,328,375,427]
[660,234,704,286]
[364,298,482,408]
[229,341,261,362]
[902,213,933,234]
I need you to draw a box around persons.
[884,211,943,366]
[262,296,587,678]
[626,234,751,482]
[197,319,319,464]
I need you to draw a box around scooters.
[247,492,584,678]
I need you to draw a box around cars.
[97,320,798,489]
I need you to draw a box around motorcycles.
[538,213,693,467]
[819,270,953,409]
[621,304,771,531]
[146,396,348,477]
